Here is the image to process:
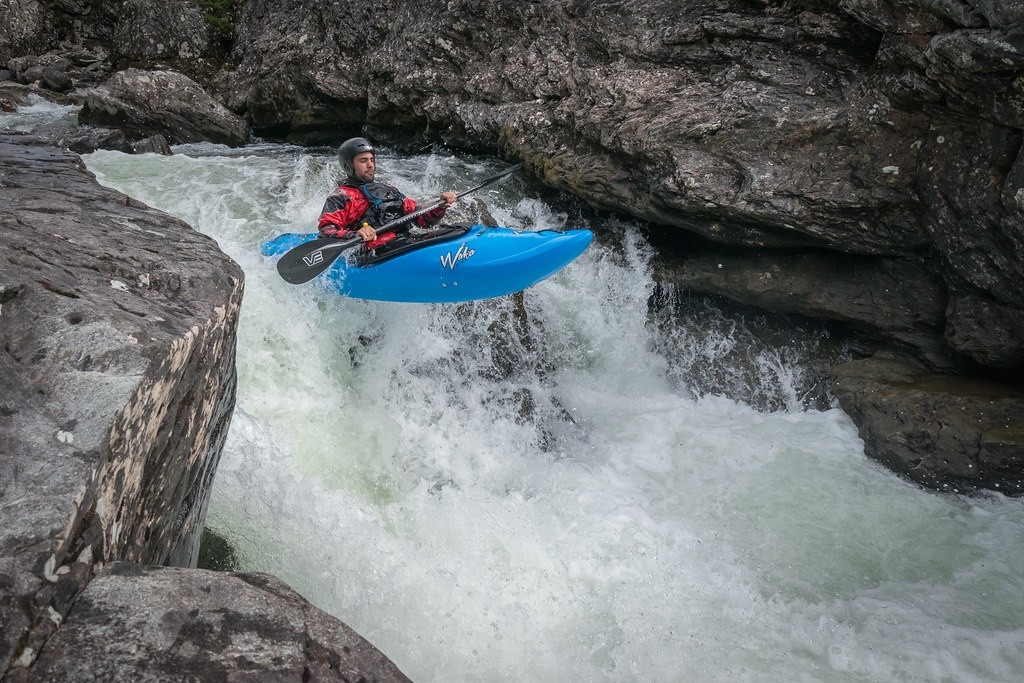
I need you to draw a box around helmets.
[338,137,376,177]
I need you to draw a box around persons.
[317,137,457,251]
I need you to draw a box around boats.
[263,225,597,305]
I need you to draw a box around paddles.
[274,159,528,286]
